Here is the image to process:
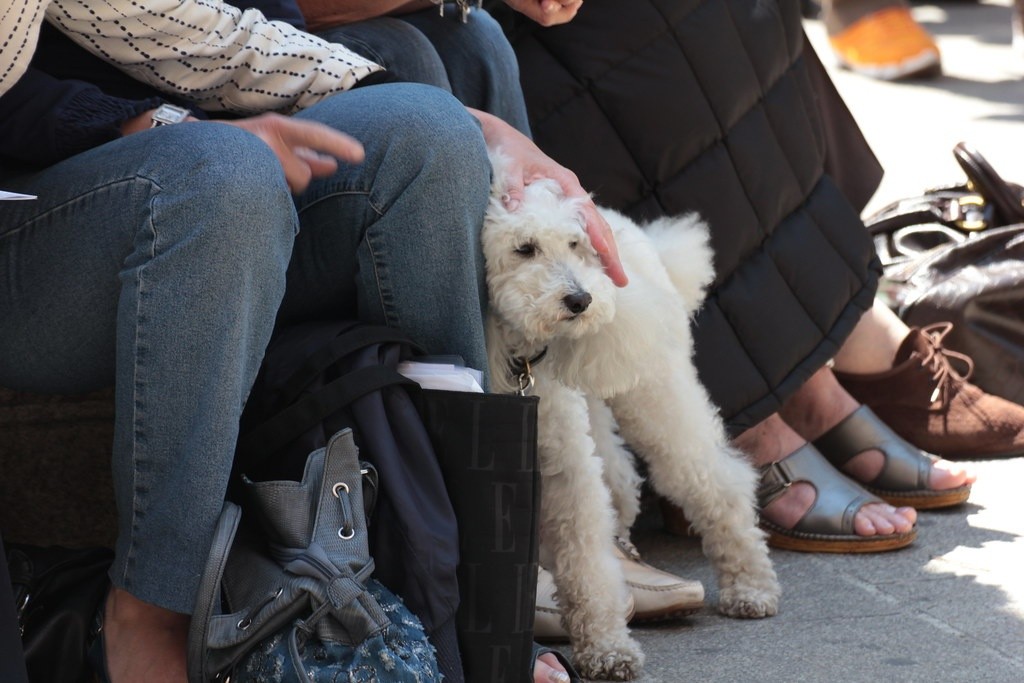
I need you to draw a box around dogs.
[481,148,783,681]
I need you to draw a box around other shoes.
[86,598,111,683]
[827,3,941,83]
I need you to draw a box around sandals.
[529,639,586,683]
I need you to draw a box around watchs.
[148,103,191,128]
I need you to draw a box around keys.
[431,0,481,24]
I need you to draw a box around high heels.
[658,441,919,553]
[810,403,971,511]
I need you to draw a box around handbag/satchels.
[235,322,544,683]
[864,143,1024,413]
[184,428,445,683]
[6,545,115,683]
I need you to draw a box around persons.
[0,0,1024,683]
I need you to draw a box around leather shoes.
[532,563,636,642]
[831,320,1024,460]
[615,534,706,623]
